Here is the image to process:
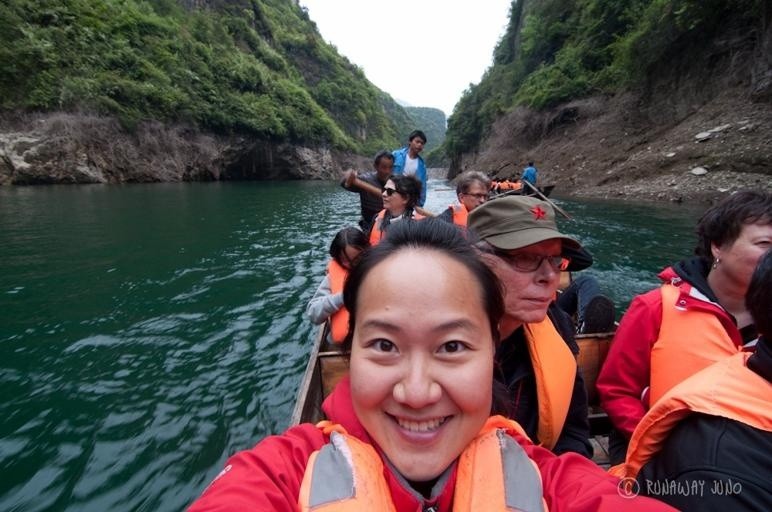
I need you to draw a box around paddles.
[522,179,572,219]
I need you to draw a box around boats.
[289,289,619,512]
[487,184,555,201]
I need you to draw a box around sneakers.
[580,295,616,334]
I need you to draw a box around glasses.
[477,244,572,272]
[466,193,491,201]
[381,186,398,197]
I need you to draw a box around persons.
[594,187,771,441]
[304,126,617,348]
[608,245,771,511]
[180,213,684,512]
[462,194,597,463]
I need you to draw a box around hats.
[467,195,593,272]
